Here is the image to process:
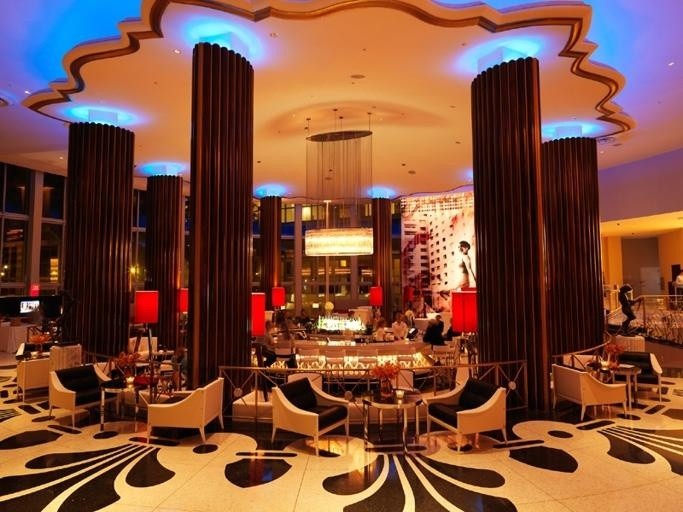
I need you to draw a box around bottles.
[317,314,362,330]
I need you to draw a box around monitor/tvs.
[19,299,40,314]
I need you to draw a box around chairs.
[16,356,52,403]
[552,364,629,423]
[148,377,224,444]
[604,349,662,403]
[614,334,645,352]
[423,378,508,452]
[49,365,116,430]
[273,379,350,455]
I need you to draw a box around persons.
[391,313,408,340]
[454,242,476,289]
[376,320,386,343]
[171,348,187,380]
[423,315,460,346]
[374,312,386,328]
[347,311,356,322]
[255,307,310,370]
[404,303,415,328]
[676,270,683,300]
[52,306,64,324]
[618,284,637,334]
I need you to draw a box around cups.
[600,360,610,371]
[30,351,38,358]
[126,377,134,384]
[395,390,404,399]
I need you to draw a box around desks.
[587,361,639,413]
[363,389,423,453]
[100,374,158,430]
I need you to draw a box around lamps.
[176,287,190,313]
[368,287,383,311]
[301,108,374,257]
[249,292,267,402]
[270,286,285,308]
[450,291,479,335]
[129,289,158,359]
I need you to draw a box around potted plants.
[29,333,50,355]
[113,352,140,385]
[604,344,621,368]
[374,362,399,398]
